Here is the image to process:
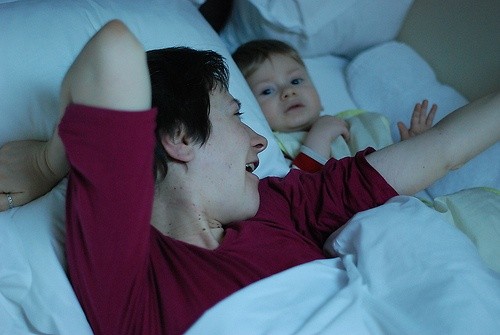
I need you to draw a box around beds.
[0,1,500,335]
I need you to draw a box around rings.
[5,193,14,209]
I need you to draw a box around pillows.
[220,1,413,60]
[0,0,290,335]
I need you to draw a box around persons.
[230,38,439,171]
[0,19,500,335]
